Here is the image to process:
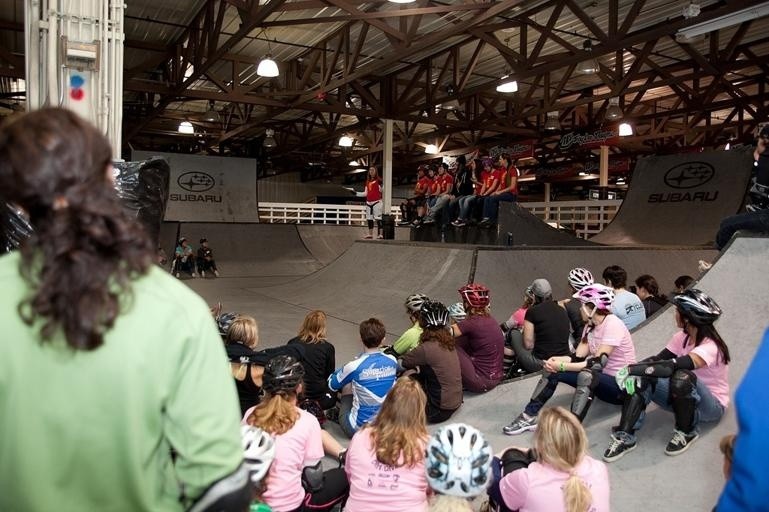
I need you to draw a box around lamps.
[496,37,518,92]
[576,16,601,74]
[262,125,278,147]
[604,92,624,122]
[255,50,280,81]
[200,100,220,122]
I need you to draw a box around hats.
[530,278,552,297]
[456,155,466,163]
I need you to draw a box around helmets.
[405,284,490,328]
[572,284,613,313]
[218,312,239,336]
[482,159,494,166]
[668,289,722,326]
[425,424,492,497]
[262,355,304,394]
[568,268,594,290]
[417,163,447,177]
[242,424,276,483]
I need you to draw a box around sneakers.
[664,428,698,455]
[503,413,537,434]
[397,217,494,232]
[603,434,637,462]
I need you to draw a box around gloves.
[615,366,642,395]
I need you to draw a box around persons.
[378,261,697,423]
[343,376,431,511]
[1,104,243,512]
[241,424,276,512]
[503,282,636,435]
[716,122,769,251]
[238,355,349,511]
[175,236,197,278]
[154,246,167,267]
[209,302,242,344]
[197,237,220,278]
[425,406,612,511]
[349,165,384,239]
[602,288,731,462]
[225,315,349,464]
[328,318,401,440]
[397,152,522,231]
[712,329,769,512]
